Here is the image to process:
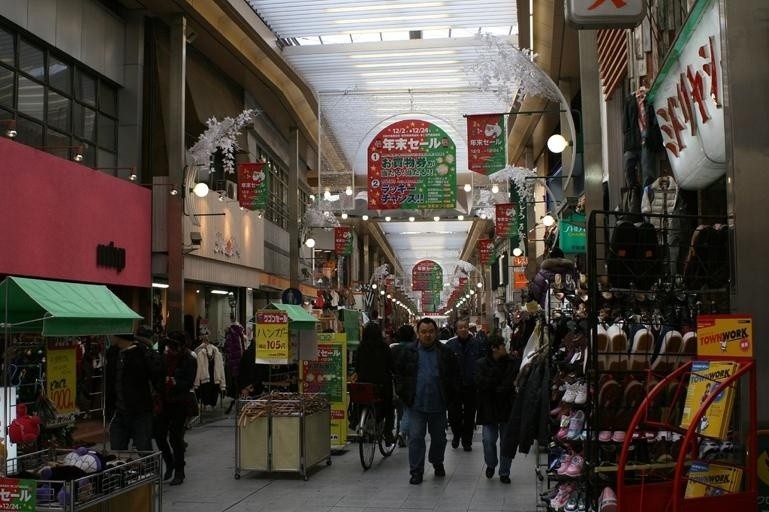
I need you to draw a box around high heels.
[552,271,719,331]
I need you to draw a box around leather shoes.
[486,459,511,484]
[410,462,445,484]
[608,220,729,290]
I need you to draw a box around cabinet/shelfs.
[534,210,737,512]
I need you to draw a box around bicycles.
[358,382,401,470]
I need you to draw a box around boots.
[164,441,186,485]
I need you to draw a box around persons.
[105,324,198,486]
[351,318,521,485]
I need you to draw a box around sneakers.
[540,323,734,511]
[452,434,472,451]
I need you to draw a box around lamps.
[543,215,557,226]
[303,239,315,247]
[547,134,573,153]
[513,248,524,256]
[188,183,209,198]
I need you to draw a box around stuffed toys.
[36,446,102,504]
[9,403,42,444]
